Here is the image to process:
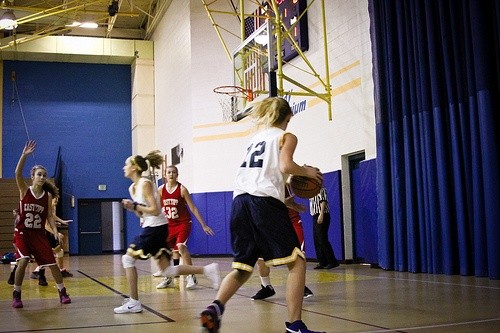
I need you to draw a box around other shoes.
[314,262,327,269]
[324,261,339,269]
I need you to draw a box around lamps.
[254,30,268,45]
[0,9,18,30]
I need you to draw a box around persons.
[249,182,314,300]
[6,195,73,286]
[114,150,221,314]
[308,185,340,270]
[153,252,180,278]
[199,96,327,333]
[12,139,71,308]
[156,166,214,290]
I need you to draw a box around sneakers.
[60,269,73,277]
[157,277,174,289]
[201,300,225,333]
[285,319,326,333]
[203,263,220,290]
[12,289,23,309]
[57,287,71,304]
[251,284,276,301]
[303,286,313,298]
[186,273,198,288]
[114,298,143,314]
[30,271,39,279]
[153,271,163,277]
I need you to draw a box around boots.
[37,268,48,286]
[8,264,17,285]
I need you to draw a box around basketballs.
[291,166,324,199]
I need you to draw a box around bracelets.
[133,204,137,210]
